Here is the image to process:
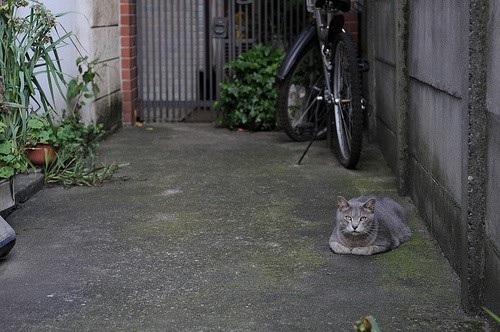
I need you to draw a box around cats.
[328,194,411,255]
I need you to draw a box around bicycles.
[276,0,374,169]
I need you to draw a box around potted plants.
[215,41,310,133]
[24,113,83,170]
